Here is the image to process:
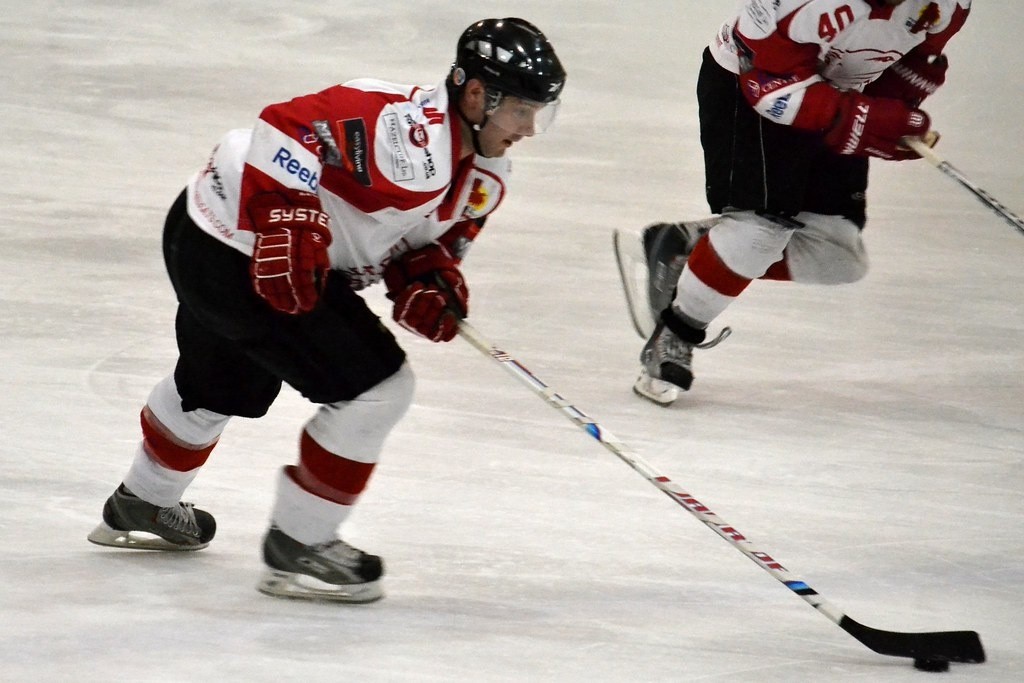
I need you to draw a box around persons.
[609,0,972,407]
[84,18,565,603]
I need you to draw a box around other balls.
[912,656,951,672]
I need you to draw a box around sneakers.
[633,287,734,408]
[612,222,707,340]
[88,481,217,551]
[255,520,386,602]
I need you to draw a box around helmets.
[452,17,565,104]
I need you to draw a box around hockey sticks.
[456,319,989,665]
[907,136,1023,230]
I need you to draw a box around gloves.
[246,188,334,314]
[824,89,939,160]
[864,51,949,106]
[383,242,468,343]
